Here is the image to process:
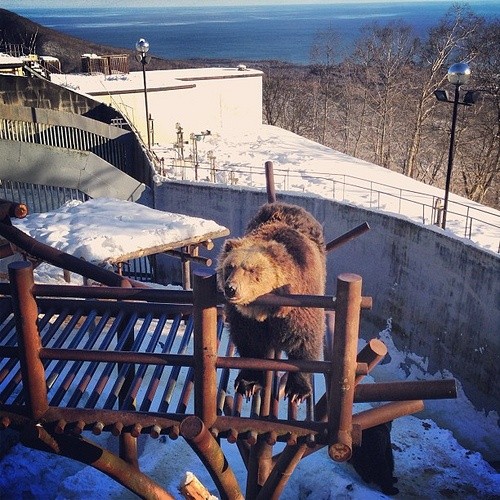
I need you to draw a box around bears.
[214,200,328,407]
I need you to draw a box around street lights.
[133,37,152,152]
[431,62,480,230]
